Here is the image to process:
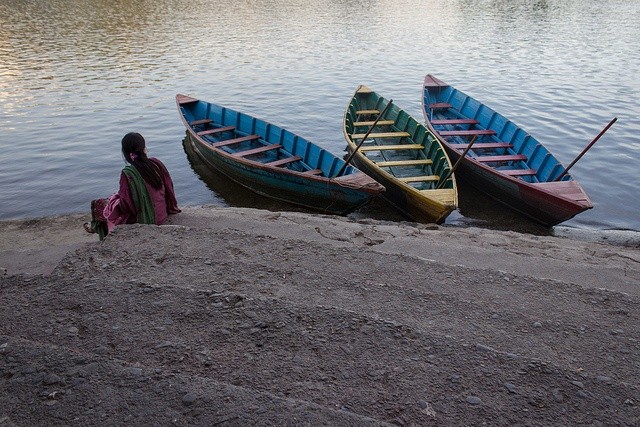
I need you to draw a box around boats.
[176,94,387,214]
[422,74,594,227]
[343,86,458,223]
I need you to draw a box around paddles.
[333,99,394,177]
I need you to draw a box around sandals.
[83,222,95,233]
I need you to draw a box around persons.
[82,132,180,234]
